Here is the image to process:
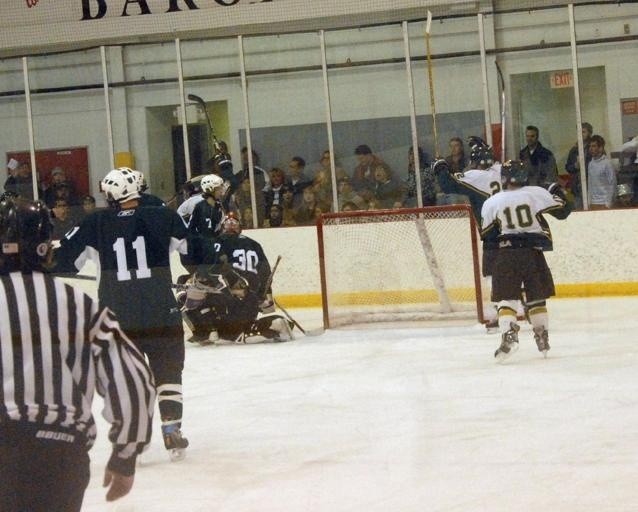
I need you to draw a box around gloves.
[431,157,449,172]
[542,180,561,196]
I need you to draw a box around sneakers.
[495,332,521,360]
[160,421,189,450]
[269,315,292,339]
[534,332,551,351]
[187,329,218,342]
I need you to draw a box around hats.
[52,167,64,175]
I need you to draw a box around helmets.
[201,174,224,193]
[220,216,241,234]
[502,160,531,188]
[1,194,57,274]
[470,143,496,166]
[99,167,148,211]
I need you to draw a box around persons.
[432,134,528,329]
[3,124,638,239]
[182,211,295,346]
[48,166,251,449]
[176,174,226,331]
[480,158,573,359]
[1,197,157,512]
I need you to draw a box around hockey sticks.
[425,10,440,160]
[188,94,219,156]
[496,61,506,164]
[274,300,325,336]
